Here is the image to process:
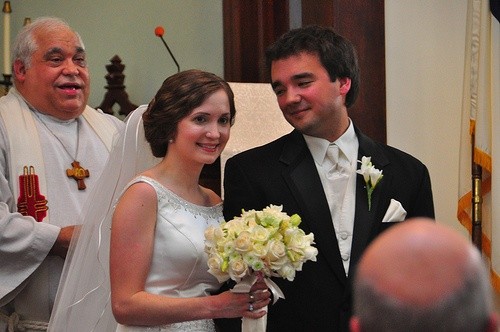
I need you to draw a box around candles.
[2,1,12,76]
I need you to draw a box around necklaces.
[21,97,90,191]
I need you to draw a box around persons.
[350,218,500,332]
[0,17,125,332]
[223,24,435,332]
[46,69,271,332]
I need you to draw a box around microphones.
[154,26,180,72]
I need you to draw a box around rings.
[249,303,253,311]
[249,293,254,303]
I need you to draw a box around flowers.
[355,155,383,211]
[204,204,319,332]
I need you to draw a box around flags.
[455,0,500,296]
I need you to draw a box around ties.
[325,144,351,224]
